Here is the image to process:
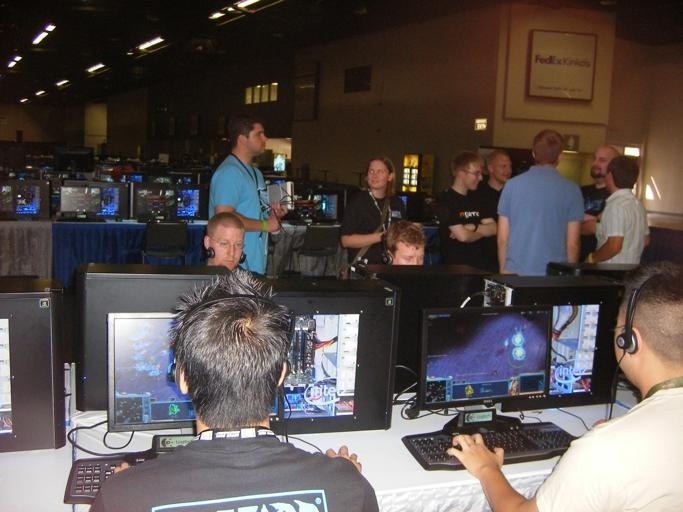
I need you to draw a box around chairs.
[288,224,341,275]
[139,222,192,267]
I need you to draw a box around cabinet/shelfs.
[400,152,432,195]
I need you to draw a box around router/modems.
[266,182,295,210]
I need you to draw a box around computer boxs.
[0,276,66,452]
[306,190,341,222]
[482,275,627,414]
[16,179,51,221]
[88,181,128,220]
[62,180,89,217]
[176,181,209,218]
[349,261,494,393]
[269,276,402,435]
[130,181,140,220]
[545,261,644,281]
[76,262,232,411]
[40,170,72,195]
[128,172,148,184]
[169,170,193,186]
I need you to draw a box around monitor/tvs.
[396,193,410,215]
[107,312,284,432]
[0,180,17,214]
[417,303,553,433]
[53,145,95,179]
[59,185,102,214]
[100,172,127,183]
[271,177,289,185]
[132,181,178,220]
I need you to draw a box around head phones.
[380,237,395,265]
[171,293,296,383]
[206,245,247,264]
[173,178,184,203]
[616,271,665,354]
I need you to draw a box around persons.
[203,212,264,277]
[88,269,378,511]
[496,129,584,276]
[434,150,497,267]
[381,221,424,265]
[579,145,619,264]
[581,155,652,263]
[339,155,407,280]
[207,117,287,277]
[470,150,512,257]
[447,262,683,510]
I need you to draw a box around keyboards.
[137,218,177,223]
[56,213,105,222]
[0,216,18,221]
[401,408,576,471]
[138,214,177,223]
[63,434,196,504]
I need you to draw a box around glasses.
[208,234,244,248]
[460,168,482,176]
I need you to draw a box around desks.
[0,355,641,512]
[1,213,441,293]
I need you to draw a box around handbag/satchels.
[337,196,394,280]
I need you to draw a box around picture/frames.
[526,28,600,102]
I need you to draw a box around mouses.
[30,216,40,222]
[115,216,123,222]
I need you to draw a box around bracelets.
[260,219,267,231]
[472,224,478,232]
[587,252,592,264]
